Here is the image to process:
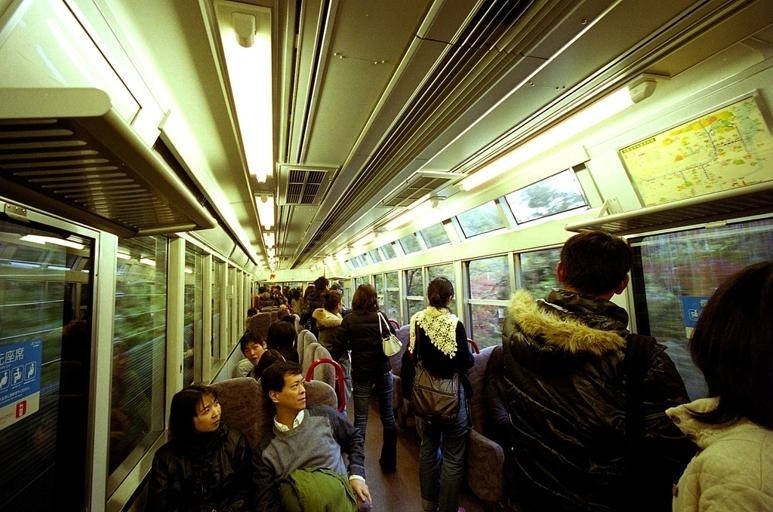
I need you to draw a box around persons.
[665,262,772,511]
[478,232,698,511]
[145,274,477,512]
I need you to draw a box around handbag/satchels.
[382,333,402,356]
[412,365,461,421]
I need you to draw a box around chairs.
[206,306,506,502]
